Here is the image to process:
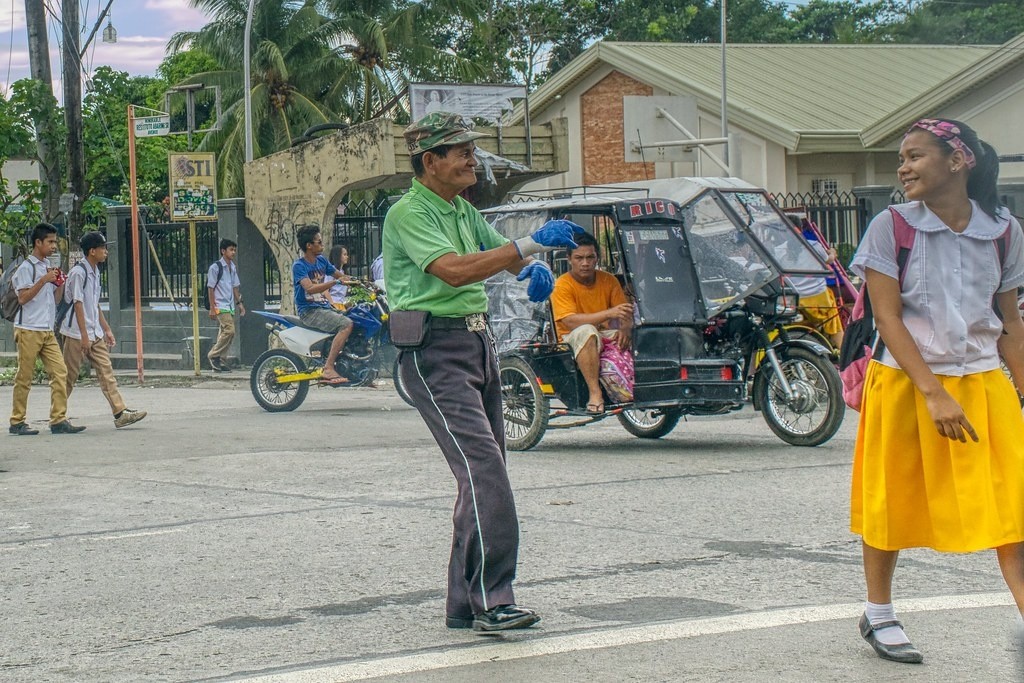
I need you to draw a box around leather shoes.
[474,604,542,632]
[447,615,473,628]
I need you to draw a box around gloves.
[516,259,556,303]
[514,219,585,260]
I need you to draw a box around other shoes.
[859,611,922,661]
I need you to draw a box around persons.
[549,229,642,414]
[0,222,87,435]
[775,214,846,351]
[58,232,147,429]
[207,240,246,373]
[381,112,586,630]
[852,116,1023,666]
[293,225,354,383]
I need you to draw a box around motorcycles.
[248,276,421,412]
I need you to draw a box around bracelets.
[237,299,243,304]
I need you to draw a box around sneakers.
[211,366,232,373]
[50,420,86,434]
[8,421,39,435]
[209,356,221,371]
[115,411,147,429]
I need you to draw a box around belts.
[429,313,487,332]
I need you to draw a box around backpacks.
[0,256,36,321]
[840,205,1013,411]
[595,336,634,404]
[56,262,88,322]
[203,261,223,310]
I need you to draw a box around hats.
[403,110,491,157]
[81,232,117,251]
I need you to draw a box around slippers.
[320,377,350,383]
[585,402,605,415]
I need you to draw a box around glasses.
[310,239,323,246]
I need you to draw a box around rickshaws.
[473,174,848,454]
